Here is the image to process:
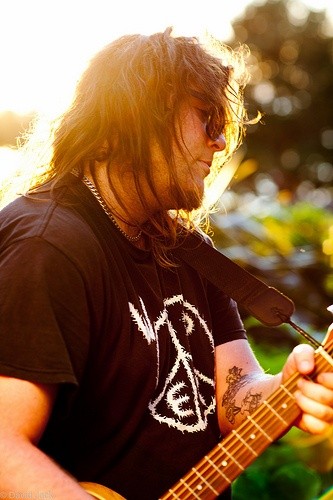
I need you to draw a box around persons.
[1,26,333,500]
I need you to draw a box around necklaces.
[71,167,142,241]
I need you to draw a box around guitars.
[74,324,333,500]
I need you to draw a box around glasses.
[173,84,226,141]
